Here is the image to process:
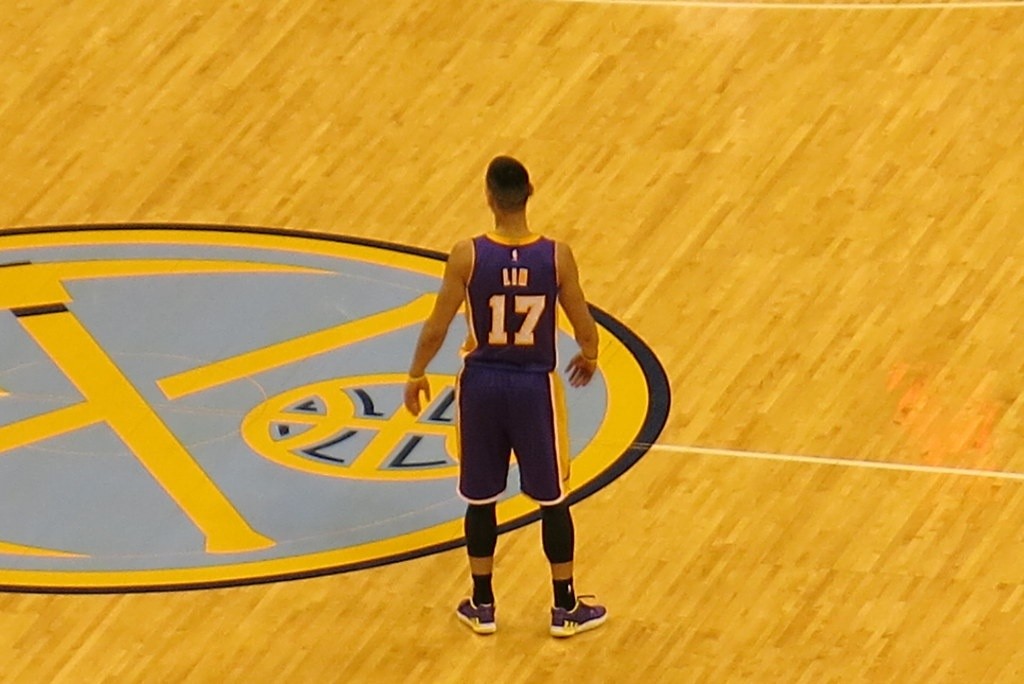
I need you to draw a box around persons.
[405,156,608,637]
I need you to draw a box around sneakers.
[457,595,496,635]
[549,594,607,637]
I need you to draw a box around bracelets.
[410,374,426,383]
[579,351,597,363]
